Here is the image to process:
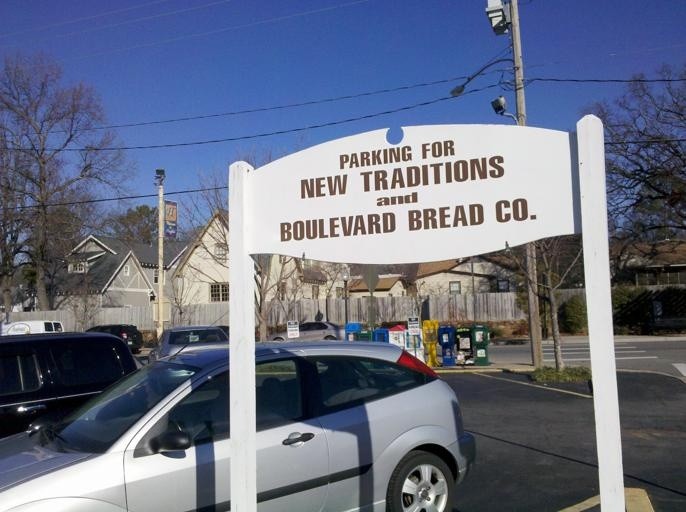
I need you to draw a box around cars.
[267,321,346,342]
[148,326,229,365]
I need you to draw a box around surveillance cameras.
[491,95,506,114]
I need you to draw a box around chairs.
[262,378,282,406]
[322,361,358,405]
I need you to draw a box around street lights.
[452,1,546,368]
[150,167,165,340]
[338,264,351,340]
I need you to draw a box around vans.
[0,320,65,337]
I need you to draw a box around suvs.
[86,324,144,355]
[1,330,140,439]
[0,340,477,512]
